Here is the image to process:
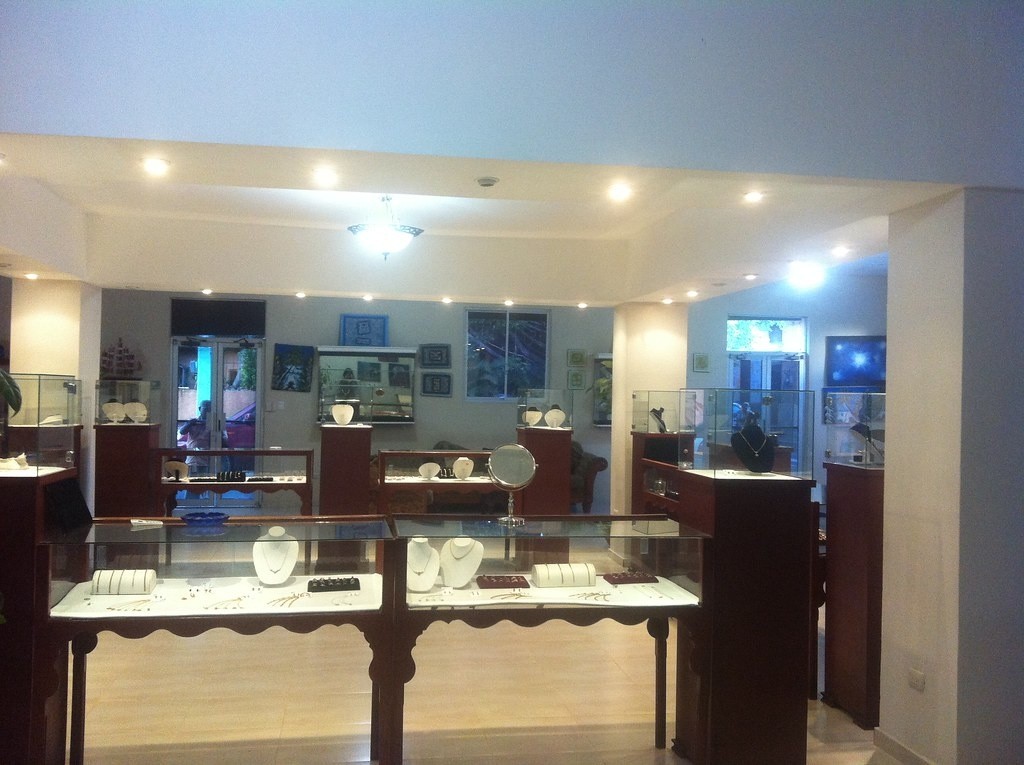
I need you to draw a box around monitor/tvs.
[823,336,886,386]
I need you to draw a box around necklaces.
[96,568,151,596]
[739,430,767,457]
[261,541,290,573]
[408,543,432,574]
[449,539,479,560]
[545,561,592,585]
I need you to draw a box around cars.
[177,401,255,471]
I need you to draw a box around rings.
[312,576,356,587]
[612,571,653,581]
[482,574,525,584]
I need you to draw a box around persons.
[179,400,228,498]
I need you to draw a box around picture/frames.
[420,372,453,397]
[419,344,451,368]
[568,370,585,390]
[567,349,587,366]
[693,353,711,373]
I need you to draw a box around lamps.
[347,198,424,262]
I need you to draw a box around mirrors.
[485,445,539,526]
[317,354,417,420]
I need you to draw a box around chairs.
[571,441,609,513]
